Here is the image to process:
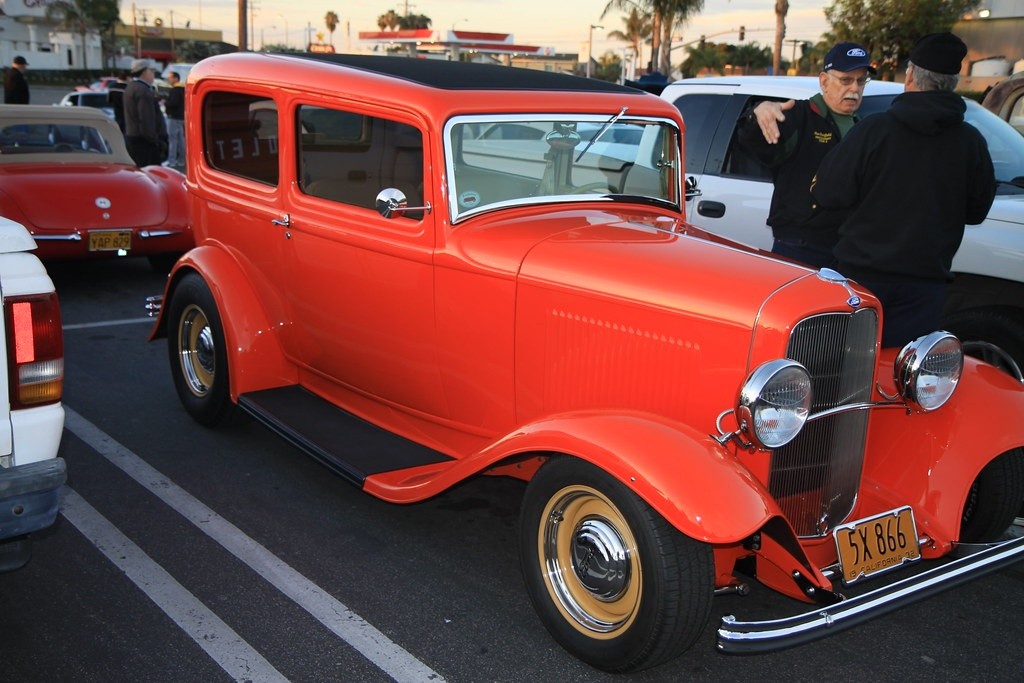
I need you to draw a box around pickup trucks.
[459,71,1024,381]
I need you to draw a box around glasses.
[827,71,870,86]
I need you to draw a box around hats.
[13,55,29,66]
[824,41,877,76]
[131,59,156,73]
[903,32,967,75]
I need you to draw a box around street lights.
[586,24,605,79]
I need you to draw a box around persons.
[734,42,876,272]
[3,55,32,105]
[160,72,187,169]
[122,58,170,166]
[807,29,998,353]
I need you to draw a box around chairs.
[304,146,502,220]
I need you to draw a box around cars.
[976,69,1023,136]
[0,100,196,286]
[142,56,1024,672]
[0,209,69,575]
[246,99,365,140]
[47,62,199,155]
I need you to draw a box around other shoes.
[176,160,186,167]
[161,161,176,167]
